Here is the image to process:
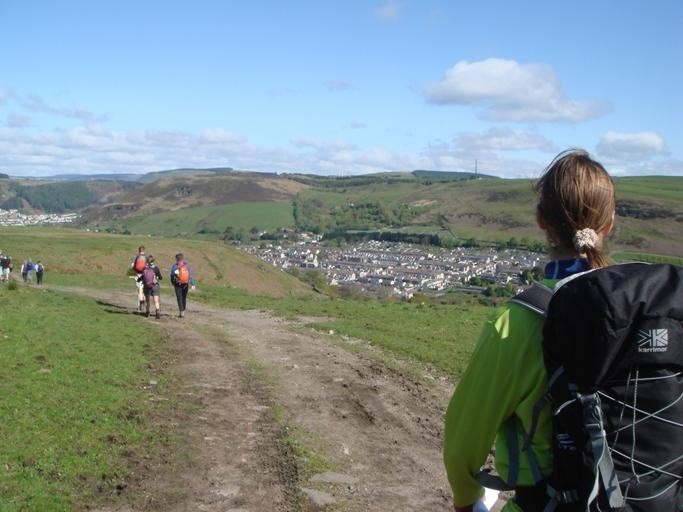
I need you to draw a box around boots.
[155,309,160,320]
[144,311,150,318]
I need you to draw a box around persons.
[171,254,192,317]
[131,246,147,311]
[137,255,162,320]
[0,254,44,285]
[444,147,615,512]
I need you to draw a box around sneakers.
[136,301,144,311]
[177,310,186,320]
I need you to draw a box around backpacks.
[503,260,682,512]
[176,264,189,285]
[0,257,11,270]
[142,267,157,290]
[23,262,43,277]
[132,254,146,273]
